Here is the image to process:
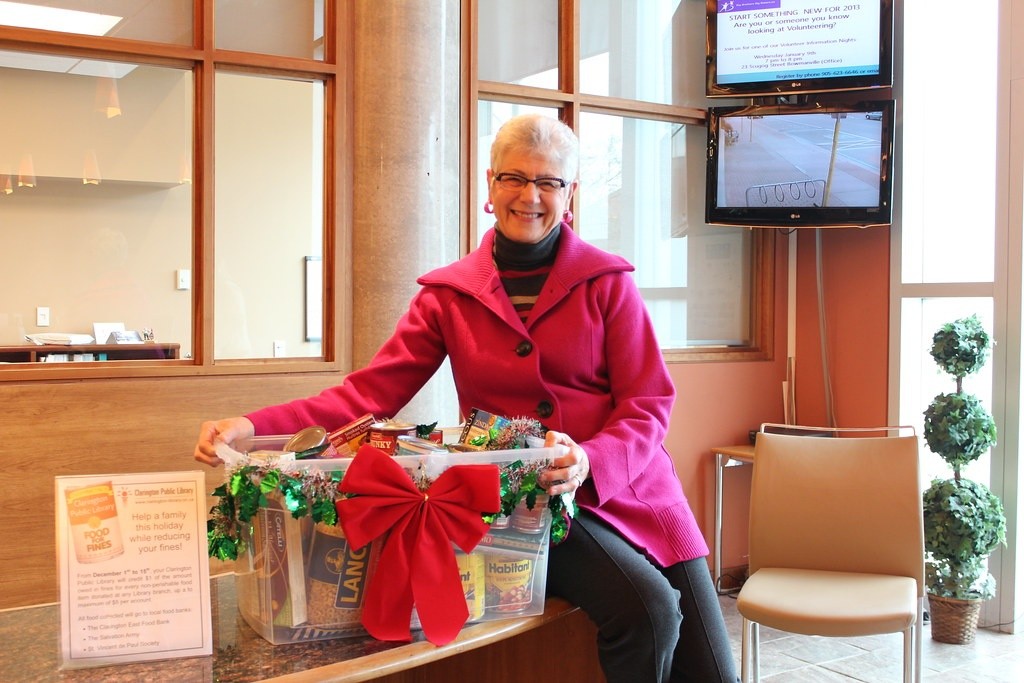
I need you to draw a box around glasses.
[492,171,575,192]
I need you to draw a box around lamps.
[1,68,121,195]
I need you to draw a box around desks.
[0,342,180,363]
[711,445,758,593]
[0,571,611,682]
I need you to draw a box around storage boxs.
[214,435,570,644]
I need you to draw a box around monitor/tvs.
[703,98,895,228]
[705,0,894,98]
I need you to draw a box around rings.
[575,474,585,488]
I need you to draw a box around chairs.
[737,422,926,683]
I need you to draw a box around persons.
[195,116,742,683]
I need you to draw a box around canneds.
[452,486,551,620]
[305,517,385,631]
[280,424,453,461]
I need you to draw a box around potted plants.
[924,313,1007,644]
